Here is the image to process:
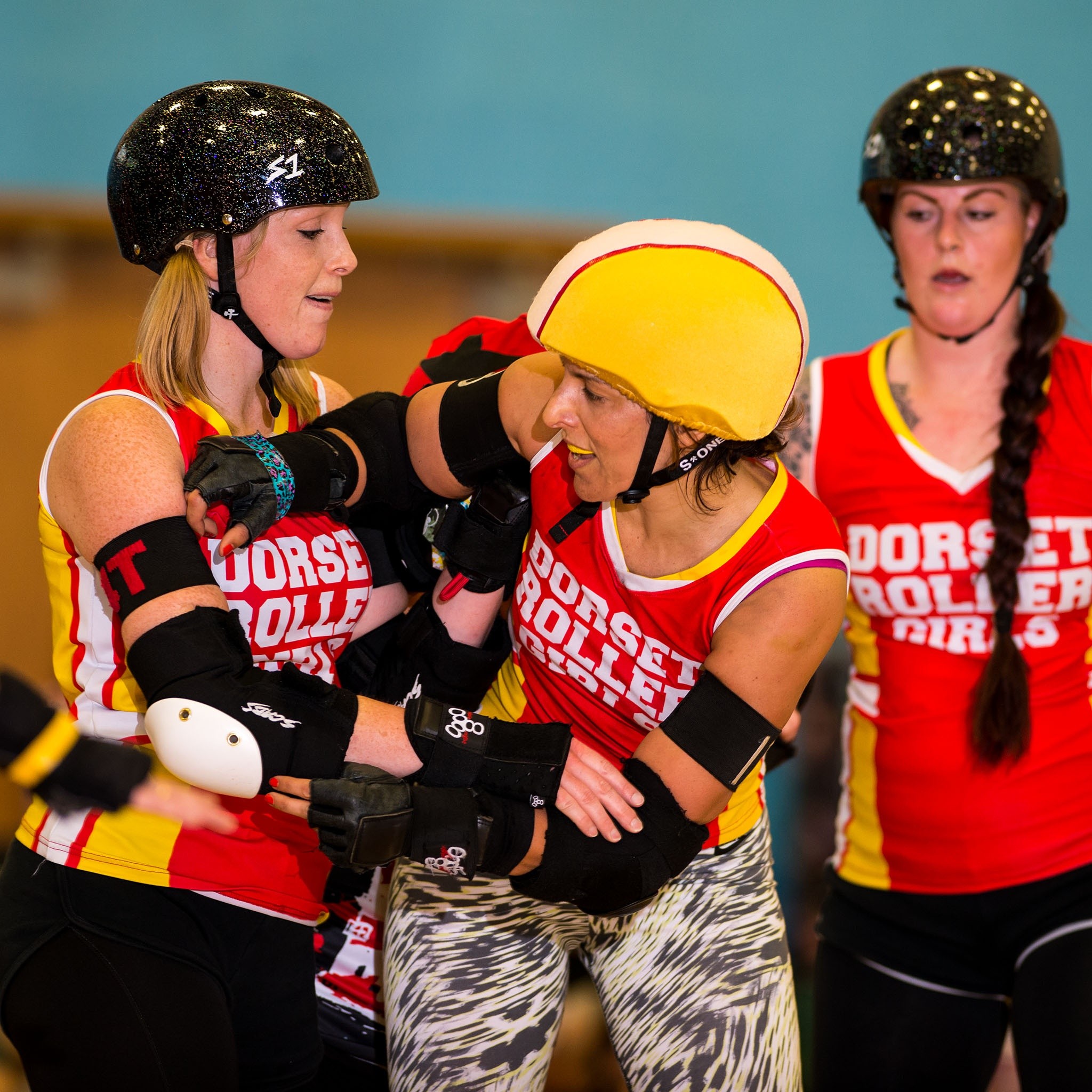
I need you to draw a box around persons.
[760,64,1092,1092]
[0,82,644,1092]
[180,217,849,1092]
[0,659,237,830]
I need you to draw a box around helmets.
[860,67,1069,234]
[107,81,379,267]
[526,218,811,442]
